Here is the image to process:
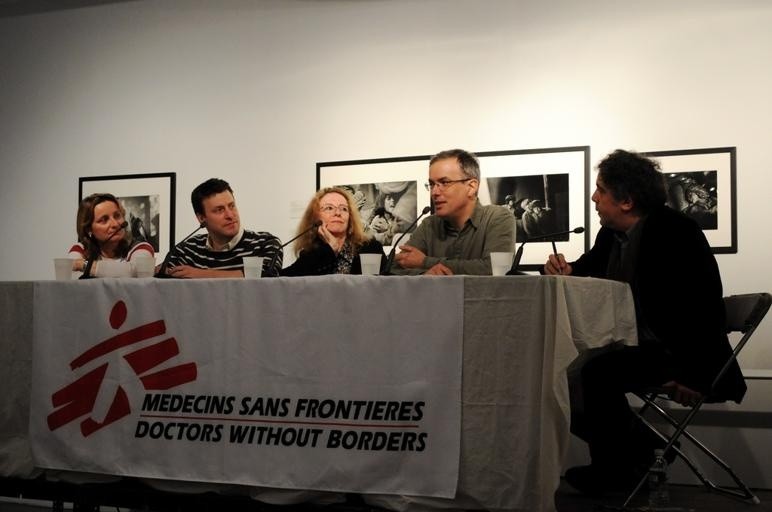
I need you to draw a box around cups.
[359,253,383,275]
[488,251,515,277]
[242,255,264,278]
[134,254,155,279]
[53,259,73,281]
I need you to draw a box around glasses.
[425,178,472,191]
[321,206,349,213]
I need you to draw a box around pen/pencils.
[551,237,563,275]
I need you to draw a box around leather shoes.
[564,465,651,496]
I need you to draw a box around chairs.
[618,294,772,510]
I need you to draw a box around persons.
[370,192,402,244]
[390,148,518,276]
[63,192,155,279]
[158,177,284,279]
[543,149,727,505]
[279,187,391,276]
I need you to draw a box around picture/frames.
[75,170,176,271]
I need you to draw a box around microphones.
[506,227,586,277]
[78,220,129,279]
[261,220,323,276]
[153,221,208,279]
[375,206,431,275]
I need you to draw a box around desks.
[0,276,633,511]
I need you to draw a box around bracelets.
[83,259,89,271]
